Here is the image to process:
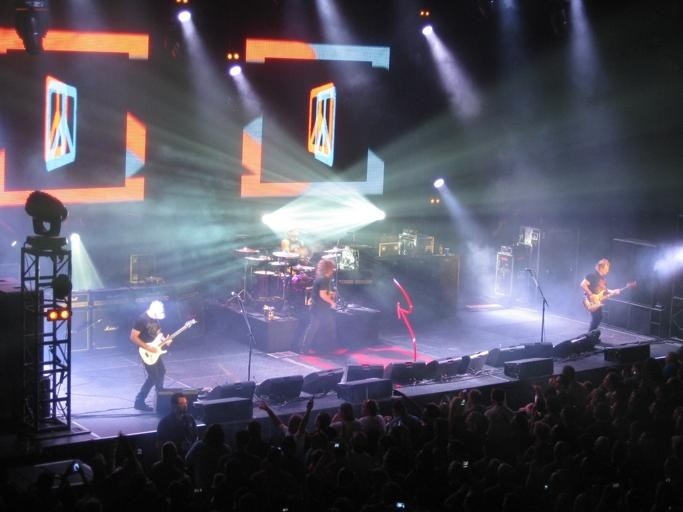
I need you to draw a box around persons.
[134,300,172,411]
[300,260,348,356]
[281,230,310,256]
[580,258,621,331]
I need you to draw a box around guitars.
[583,281,638,312]
[138,319,198,366]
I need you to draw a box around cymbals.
[245,257,266,261]
[273,253,299,257]
[324,247,343,253]
[322,255,340,258]
[236,247,260,253]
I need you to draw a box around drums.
[253,270,279,301]
[271,262,288,273]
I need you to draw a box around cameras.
[136,447,144,456]
[395,501,406,510]
[333,442,341,448]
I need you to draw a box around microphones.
[525,268,534,271]
[231,291,240,298]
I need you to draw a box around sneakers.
[133,400,154,411]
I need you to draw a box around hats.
[144,300,166,320]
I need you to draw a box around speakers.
[207,381,255,401]
[193,395,254,423]
[171,278,207,348]
[259,375,304,401]
[493,251,532,300]
[553,327,599,359]
[604,342,650,362]
[427,344,527,381]
[156,388,199,415]
[130,285,177,351]
[503,355,554,379]
[301,368,344,394]
[337,376,392,402]
[384,361,427,384]
[346,364,385,380]
[521,342,554,359]
[0,276,50,421]
[600,237,675,335]
[51,280,90,355]
[87,286,132,354]
